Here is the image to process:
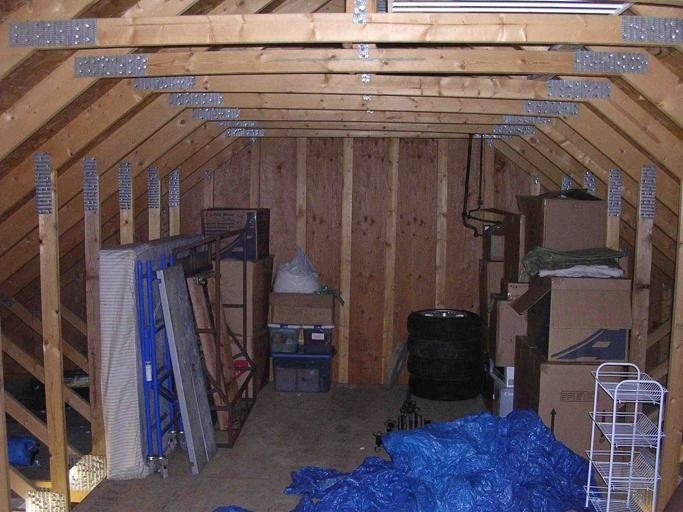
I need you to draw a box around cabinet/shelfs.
[584,361,667,510]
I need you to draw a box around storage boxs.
[202,257,268,336]
[267,323,335,357]
[478,193,632,477]
[269,292,334,326]
[200,208,270,261]
[271,353,332,392]
[198,336,273,399]
